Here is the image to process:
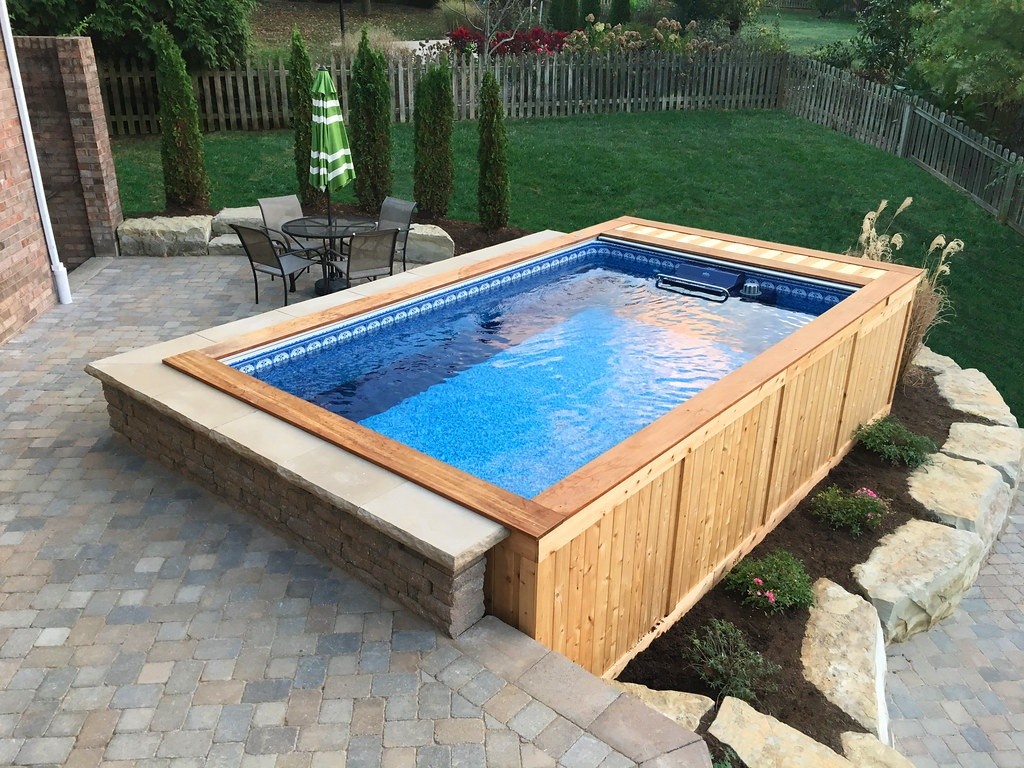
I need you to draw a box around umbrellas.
[308,65,356,281]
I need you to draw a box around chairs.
[352,197,417,283]
[330,228,401,288]
[228,223,328,307]
[257,193,339,291]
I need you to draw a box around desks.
[281,216,376,296]
